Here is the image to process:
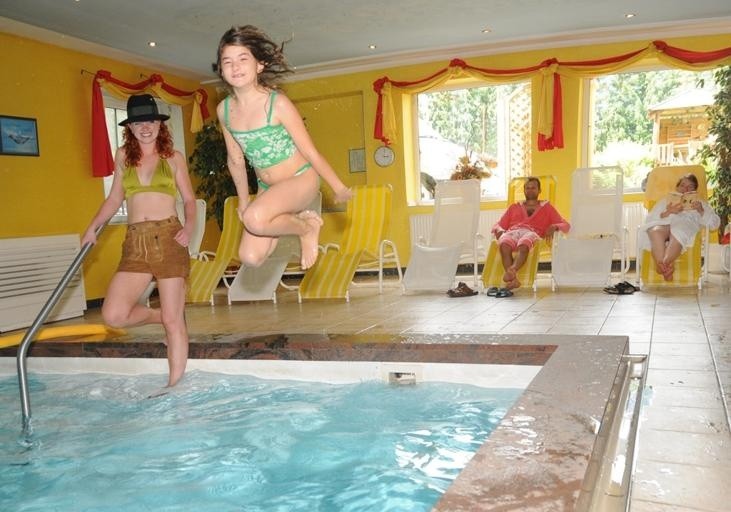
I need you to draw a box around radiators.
[410,202,708,263]
[0,233,88,333]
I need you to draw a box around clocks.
[374,146,395,166]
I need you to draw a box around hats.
[118,95,170,126]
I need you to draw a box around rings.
[184,242,186,245]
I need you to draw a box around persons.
[490,177,571,291]
[214,24,355,275]
[645,172,721,284]
[79,92,198,400]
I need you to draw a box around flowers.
[450,155,497,180]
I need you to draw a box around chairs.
[403,180,488,292]
[481,176,563,292]
[636,165,710,290]
[297,183,403,303]
[425,141,455,180]
[136,199,216,308]
[551,166,629,292]
[186,196,256,308]
[227,189,324,307]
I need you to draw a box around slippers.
[604,286,632,295]
[496,289,513,297]
[615,282,639,291]
[448,284,478,297]
[487,287,498,296]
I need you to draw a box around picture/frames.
[348,148,366,174]
[0,115,41,157]
